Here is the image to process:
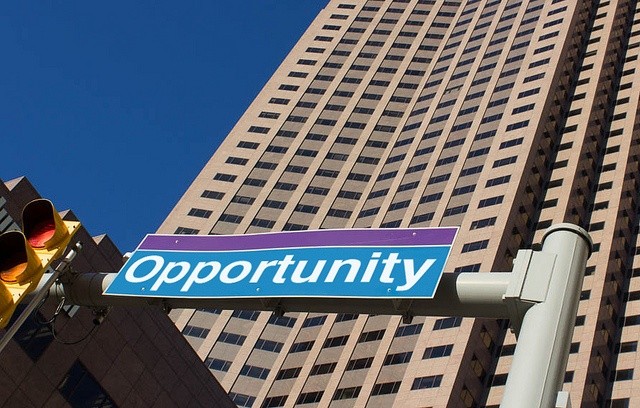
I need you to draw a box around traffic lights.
[1,198,83,330]
